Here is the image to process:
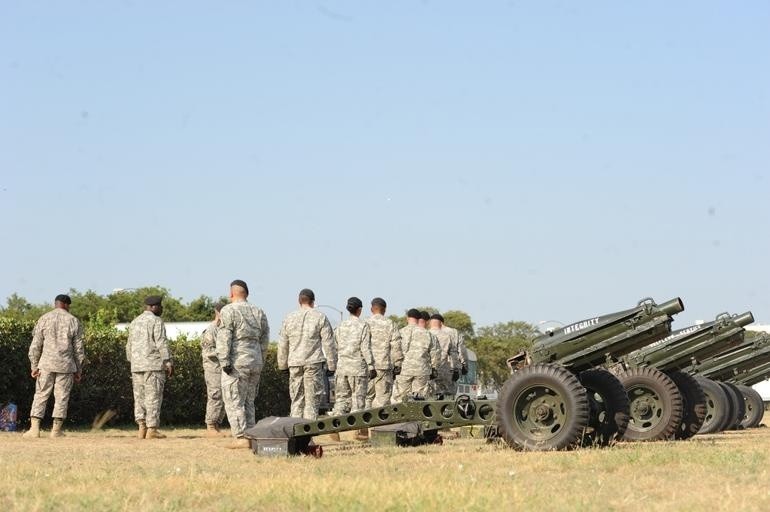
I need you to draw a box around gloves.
[167,365,174,378]
[430,369,438,380]
[223,366,232,374]
[325,370,334,377]
[393,366,401,375]
[462,365,468,375]
[369,370,377,380]
[452,369,459,382]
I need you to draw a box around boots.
[207,424,225,438]
[145,427,167,439]
[329,433,340,442]
[23,417,41,438]
[50,419,65,437]
[215,423,232,437]
[349,431,368,440]
[138,423,146,439]
[224,438,250,449]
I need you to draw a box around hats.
[144,296,162,306]
[408,309,421,319]
[419,311,430,321]
[373,298,386,307]
[431,314,444,322]
[299,288,315,300]
[55,294,71,304]
[231,280,249,295]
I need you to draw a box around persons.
[441,315,469,376]
[330,297,378,441]
[391,309,432,404]
[125,295,174,439]
[216,280,269,449]
[428,315,460,397]
[201,304,227,436]
[23,294,84,439]
[277,289,337,421]
[364,298,405,409]
[418,311,441,380]
